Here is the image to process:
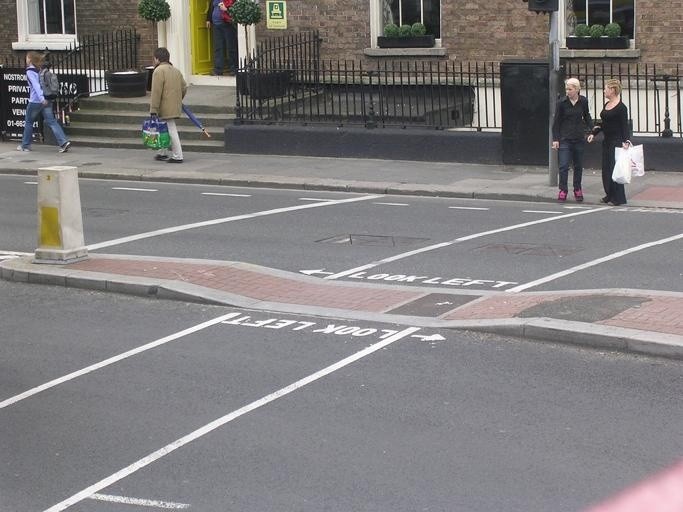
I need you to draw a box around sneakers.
[574,189,583,201]
[59,141,71,153]
[17,144,31,152]
[558,189,567,201]
[210,70,224,76]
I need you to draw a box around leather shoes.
[607,200,621,206]
[601,196,611,203]
[154,154,168,160]
[165,158,183,163]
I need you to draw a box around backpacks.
[32,66,59,99]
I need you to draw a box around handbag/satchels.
[612,139,631,184]
[141,114,171,150]
[628,139,645,177]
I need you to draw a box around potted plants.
[226,1,263,96]
[375,20,435,47]
[564,23,630,50]
[136,0,171,93]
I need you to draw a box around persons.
[149,48,187,163]
[13,51,71,153]
[586,79,630,205]
[205,1,237,76]
[550,78,601,202]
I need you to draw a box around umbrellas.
[181,102,210,138]
